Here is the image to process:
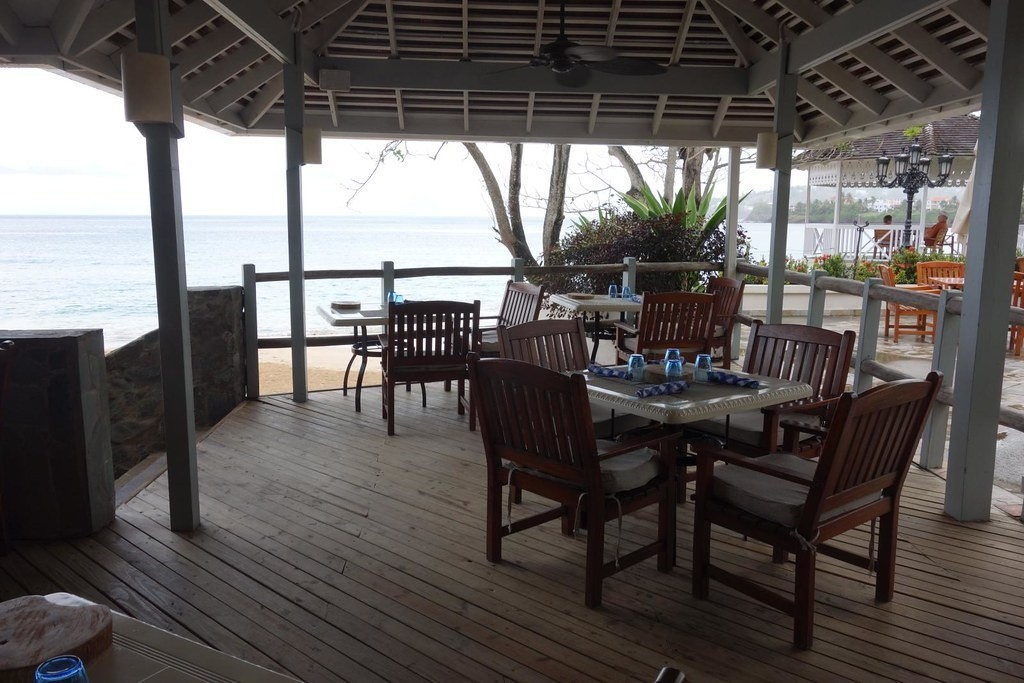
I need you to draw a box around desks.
[932,275,965,292]
[39,592,304,683]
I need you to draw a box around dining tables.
[330,302,455,414]
[549,291,674,369]
[571,356,816,564]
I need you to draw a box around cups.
[395,294,403,305]
[622,286,632,301]
[628,353,645,381]
[34,653,90,683]
[609,284,617,298]
[693,354,712,382]
[665,359,682,374]
[388,290,396,302]
[664,349,680,361]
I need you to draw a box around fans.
[469,0,669,90]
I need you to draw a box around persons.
[924,213,948,246]
[883,215,892,225]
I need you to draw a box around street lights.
[873,138,954,255]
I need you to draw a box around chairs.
[497,319,651,542]
[375,299,483,433]
[615,288,721,373]
[687,318,857,561]
[872,230,901,258]
[462,352,686,606]
[689,369,943,642]
[917,259,965,327]
[878,263,942,345]
[698,275,746,372]
[924,228,948,252]
[1007,269,1024,356]
[461,278,545,359]
